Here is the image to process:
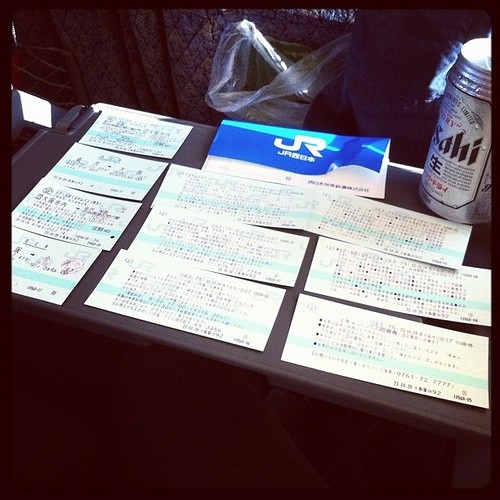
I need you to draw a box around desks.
[11,102,492,438]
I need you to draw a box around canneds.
[415,36,491,224]
